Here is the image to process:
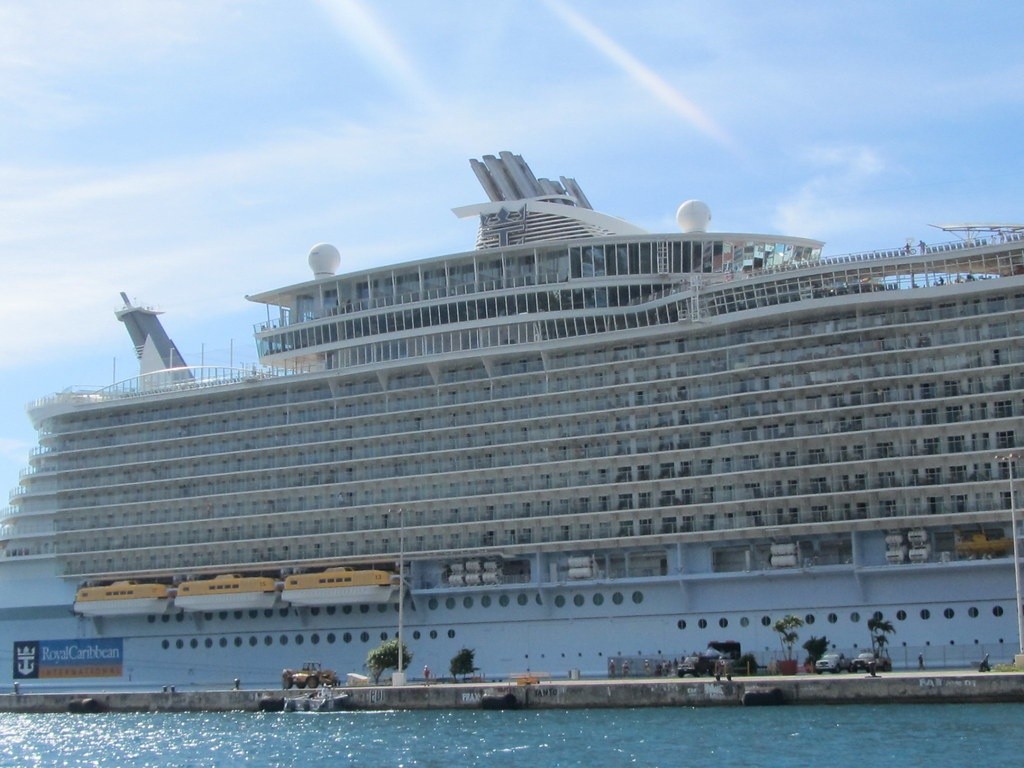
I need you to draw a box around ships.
[0,149,1024,688]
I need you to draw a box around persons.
[918,653,926,671]
[903,243,911,255]
[423,665,430,686]
[991,230,1005,245]
[932,274,999,286]
[918,240,928,255]
[609,656,687,677]
[718,656,726,675]
[282,668,290,689]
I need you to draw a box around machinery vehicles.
[292,662,340,689]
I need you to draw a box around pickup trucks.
[677,655,713,677]
[816,651,851,673]
[851,652,886,673]
